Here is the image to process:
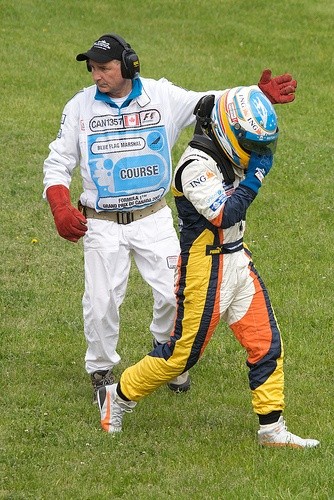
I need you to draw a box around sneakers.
[97,383,137,434]
[91,369,114,405]
[258,416,320,449]
[153,338,191,394]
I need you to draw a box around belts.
[82,197,167,225]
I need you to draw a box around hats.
[76,35,131,64]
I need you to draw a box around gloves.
[258,69,297,105]
[46,185,88,243]
[239,147,273,195]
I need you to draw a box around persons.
[43,35,299,407]
[96,85,322,448]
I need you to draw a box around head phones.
[100,34,140,80]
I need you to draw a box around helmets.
[210,85,279,170]
